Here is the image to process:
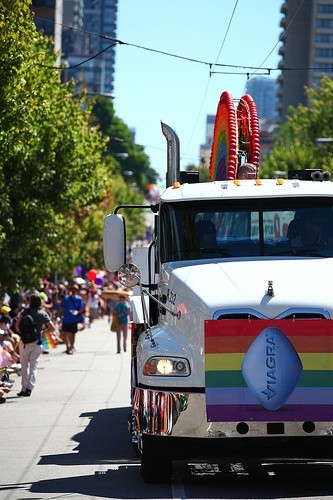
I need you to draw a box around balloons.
[88,270,103,285]
[76,266,82,276]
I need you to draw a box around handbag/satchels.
[110,314,119,332]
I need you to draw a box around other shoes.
[17,388,31,396]
[65,348,73,354]
[2,382,13,388]
[8,378,16,383]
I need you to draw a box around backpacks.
[19,310,39,346]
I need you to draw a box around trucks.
[103,120,333,485]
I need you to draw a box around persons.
[0,279,70,403]
[113,295,130,353]
[57,284,83,354]
[194,220,217,247]
[73,276,122,330]
